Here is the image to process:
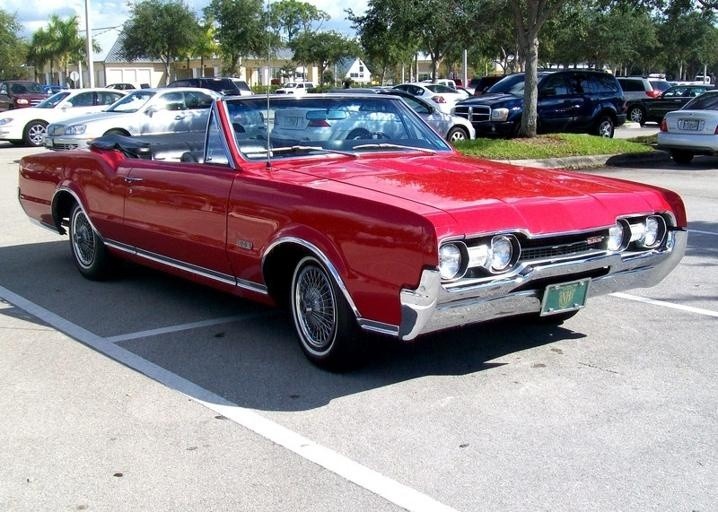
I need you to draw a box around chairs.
[144,138,274,166]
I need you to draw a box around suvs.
[450,66,631,140]
[614,73,675,114]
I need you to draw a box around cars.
[625,84,717,126]
[268,87,478,146]
[41,86,266,152]
[0,88,150,148]
[649,89,718,168]
[639,70,715,90]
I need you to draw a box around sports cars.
[16,87,693,375]
